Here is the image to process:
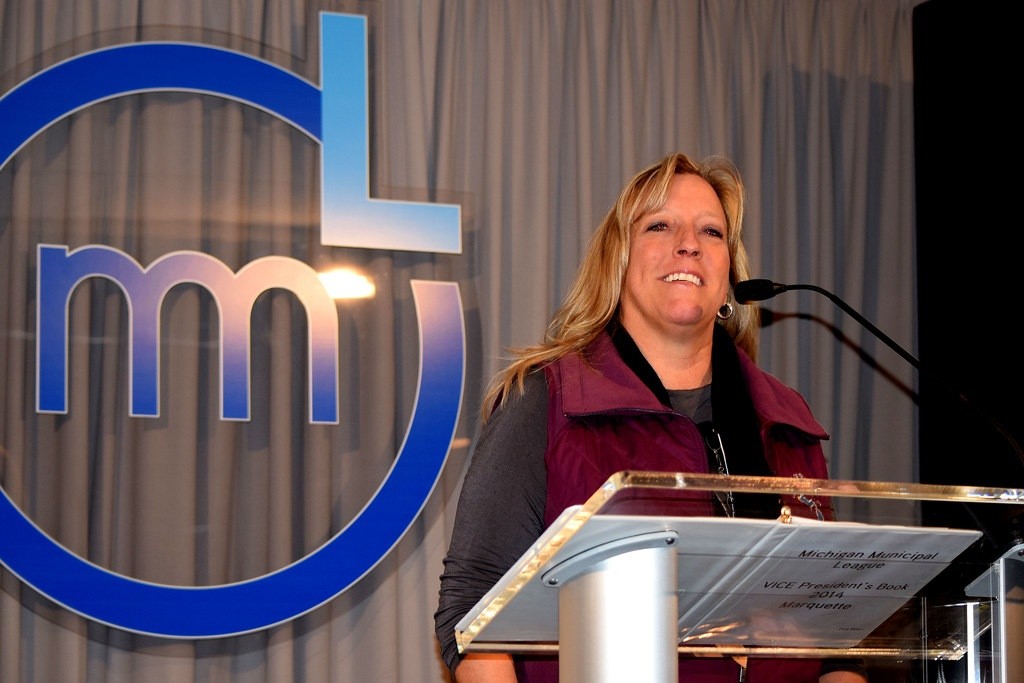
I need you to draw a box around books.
[453,504,983,648]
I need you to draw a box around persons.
[434,152,874,683]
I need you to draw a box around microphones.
[735,279,1024,465]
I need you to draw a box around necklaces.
[706,429,736,518]
